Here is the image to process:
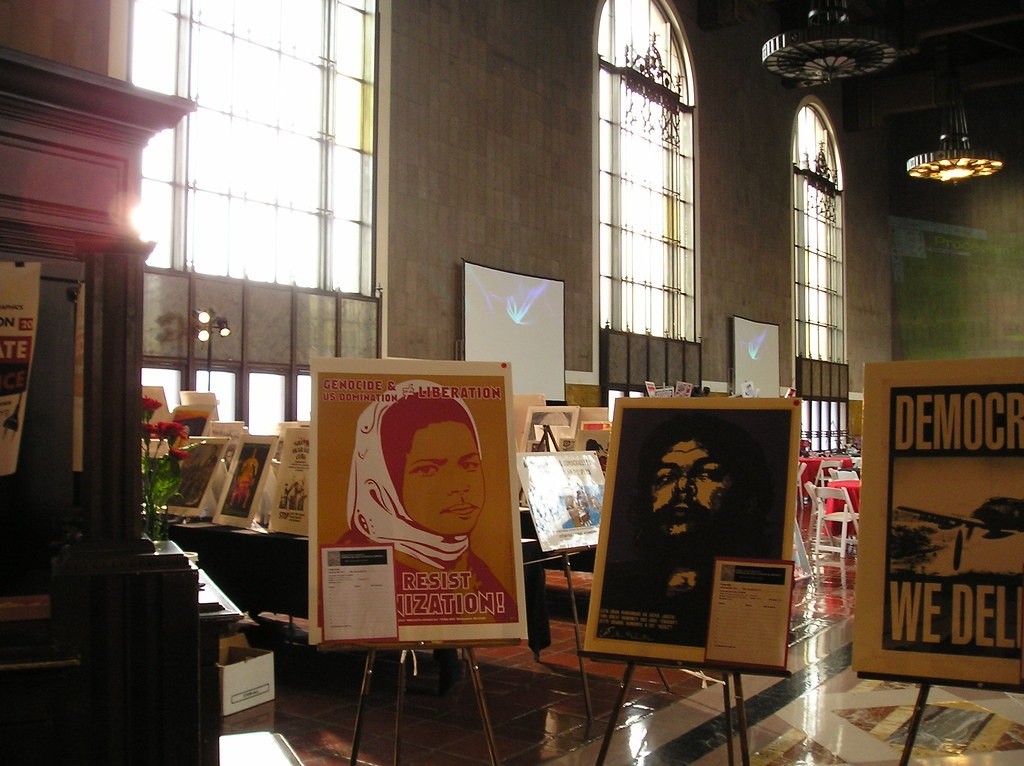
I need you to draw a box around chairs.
[816,558,856,608]
[797,455,862,558]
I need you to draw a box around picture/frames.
[142,359,528,645]
[852,352,1024,686]
[584,398,803,671]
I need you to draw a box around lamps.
[760,0,899,84]
[192,308,231,371]
[903,98,1010,187]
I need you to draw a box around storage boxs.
[218,633,276,717]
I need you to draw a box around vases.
[141,457,183,551]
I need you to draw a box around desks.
[824,480,860,545]
[799,457,854,497]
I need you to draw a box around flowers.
[141,396,193,541]
